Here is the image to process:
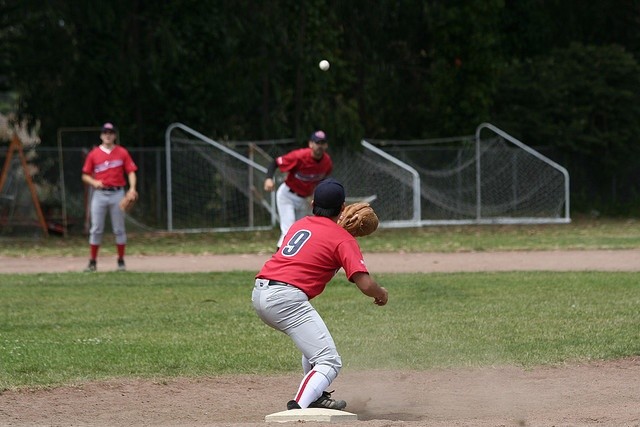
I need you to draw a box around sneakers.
[286,399,301,409]
[83,259,96,271]
[117,259,126,270]
[308,389,347,410]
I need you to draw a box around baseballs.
[319,60,330,71]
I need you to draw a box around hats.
[311,130,327,143]
[101,122,116,133]
[314,177,345,208]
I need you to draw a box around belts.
[255,280,288,286]
[289,188,305,198]
[101,185,122,190]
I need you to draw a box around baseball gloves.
[120,196,137,215]
[337,202,380,237]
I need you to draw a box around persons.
[79,121,139,271]
[248,178,390,412]
[264,130,334,262]
